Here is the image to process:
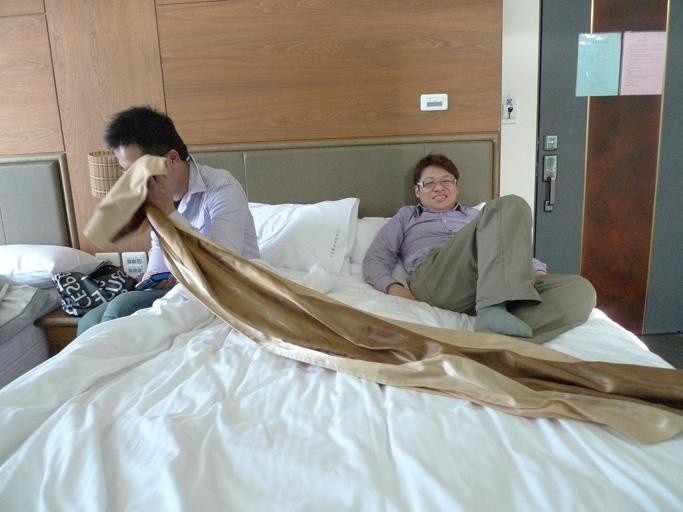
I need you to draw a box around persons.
[362,153,599,347]
[74,103,262,336]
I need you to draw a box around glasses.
[416,178,458,189]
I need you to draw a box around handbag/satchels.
[52,260,136,318]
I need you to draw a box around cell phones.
[135,272,171,290]
